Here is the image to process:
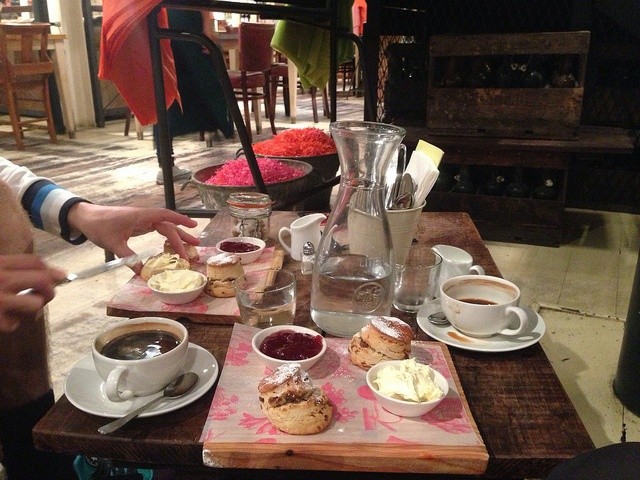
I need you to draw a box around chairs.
[1,23,56,148]
[226,22,276,144]
[272,64,328,122]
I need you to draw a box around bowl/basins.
[214,235,267,266]
[145,269,208,305]
[251,324,327,372]
[365,359,449,419]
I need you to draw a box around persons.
[1,153,201,478]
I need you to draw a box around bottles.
[309,118,409,338]
[300,241,316,276]
[227,191,273,243]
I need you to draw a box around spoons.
[429,311,450,325]
[98,371,199,434]
[387,171,418,212]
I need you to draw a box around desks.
[0,33,77,138]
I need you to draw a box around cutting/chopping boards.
[203,338,490,475]
[106,243,284,325]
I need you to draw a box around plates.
[415,297,547,353]
[64,341,219,419]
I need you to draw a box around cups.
[278,212,329,263]
[233,269,298,329]
[439,275,530,340]
[92,317,189,404]
[430,244,486,298]
[389,245,442,314]
